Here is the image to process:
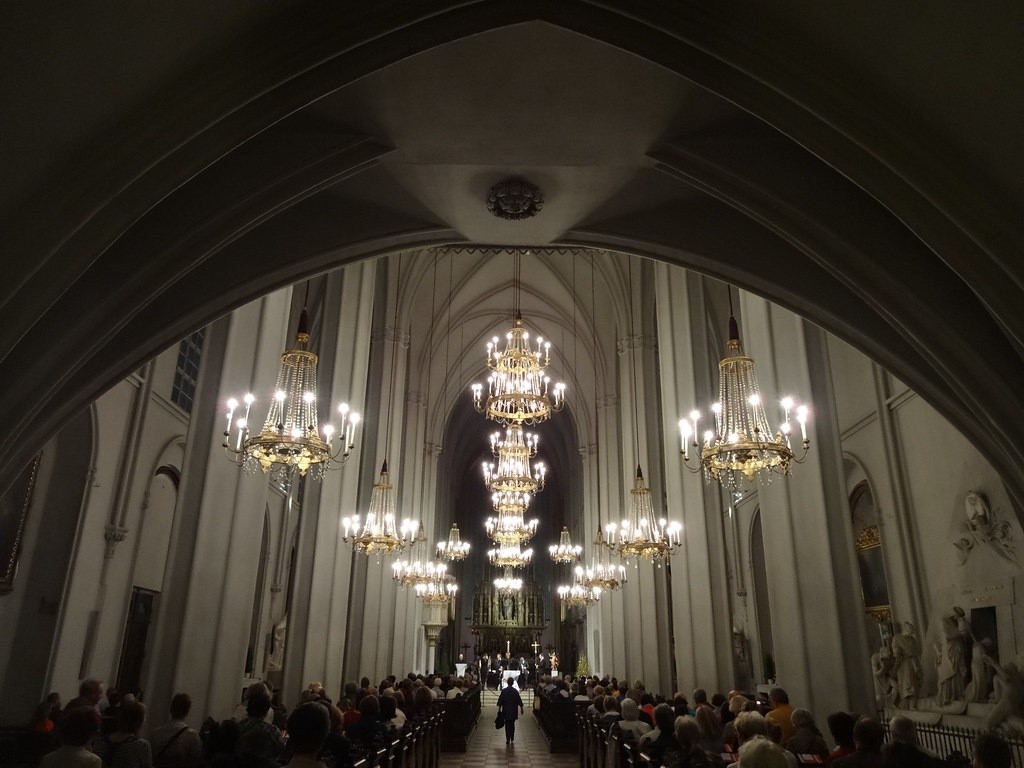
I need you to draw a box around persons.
[0,672,482,768]
[495,677,524,744]
[870,606,1024,735]
[454,651,551,690]
[539,674,1013,768]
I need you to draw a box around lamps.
[414,574,459,610]
[482,424,547,496]
[679,283,811,495]
[223,279,361,482]
[548,526,583,565]
[493,568,523,596]
[488,542,534,569]
[485,490,540,546]
[603,254,684,570]
[392,248,449,589]
[557,554,601,608]
[342,253,422,562]
[574,251,628,594]
[471,251,568,431]
[434,523,471,561]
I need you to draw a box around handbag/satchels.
[495,710,504,729]
